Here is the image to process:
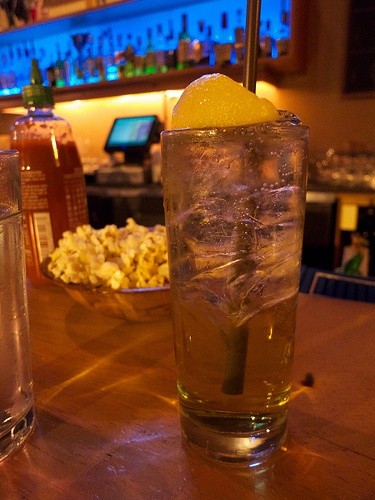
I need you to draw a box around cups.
[0,150,37,464]
[161,125,310,467]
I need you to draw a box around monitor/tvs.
[104,115,162,163]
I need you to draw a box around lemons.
[171,72,282,130]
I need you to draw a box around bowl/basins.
[40,256,172,321]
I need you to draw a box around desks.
[0,248,374,500]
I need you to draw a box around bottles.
[12,59,89,278]
[0,0,292,95]
